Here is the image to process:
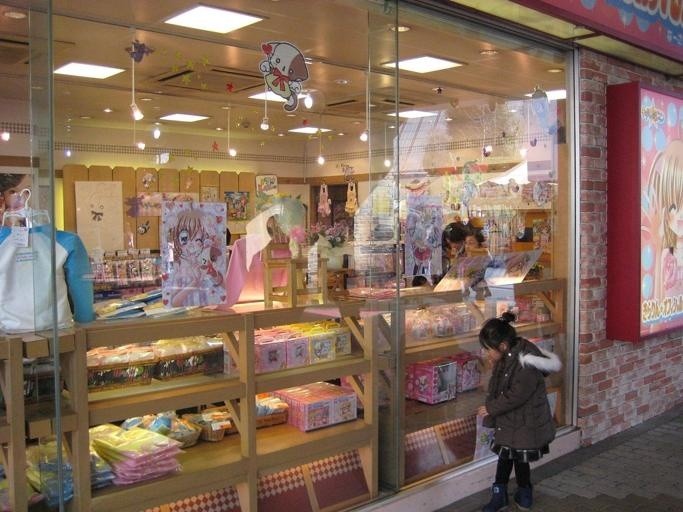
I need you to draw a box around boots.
[483,483,510,512]
[514,487,532,510]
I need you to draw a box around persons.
[477,310,562,512]
[461,225,484,253]
[442,222,466,254]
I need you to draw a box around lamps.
[258,55,270,132]
[129,24,145,121]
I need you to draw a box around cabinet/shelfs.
[439,180,559,280]
[0,273,567,507]
